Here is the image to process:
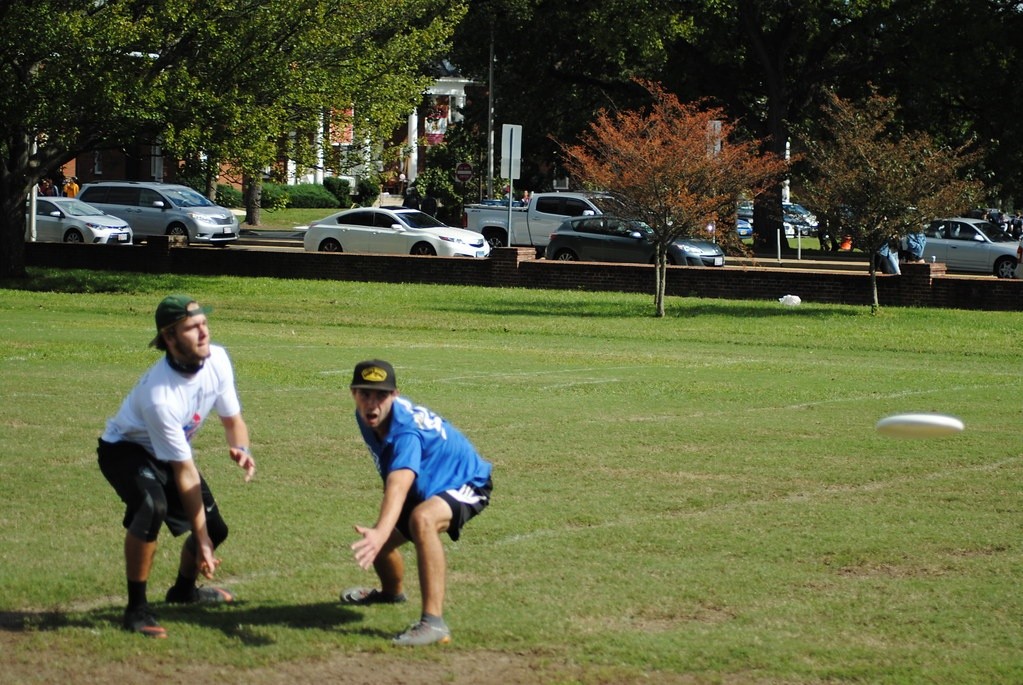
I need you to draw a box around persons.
[878,223,926,273]
[402,187,419,210]
[981,209,1023,239]
[39,179,58,196]
[444,204,464,228]
[521,191,528,205]
[340,359,492,645]
[96,294,254,637]
[63,179,79,196]
[421,190,436,216]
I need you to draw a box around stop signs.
[456,162,474,181]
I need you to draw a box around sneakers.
[165,585,236,602]
[123,614,169,639]
[390,621,452,649]
[340,587,408,606]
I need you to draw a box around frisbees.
[878,413,965,438]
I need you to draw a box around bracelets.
[239,448,250,454]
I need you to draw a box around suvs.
[545,213,726,267]
[75,179,240,248]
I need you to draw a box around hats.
[349,358,396,391]
[148,295,212,348]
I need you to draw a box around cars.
[735,200,820,239]
[23,196,134,245]
[304,205,491,259]
[920,207,1023,280]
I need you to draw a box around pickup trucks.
[461,191,630,258]
